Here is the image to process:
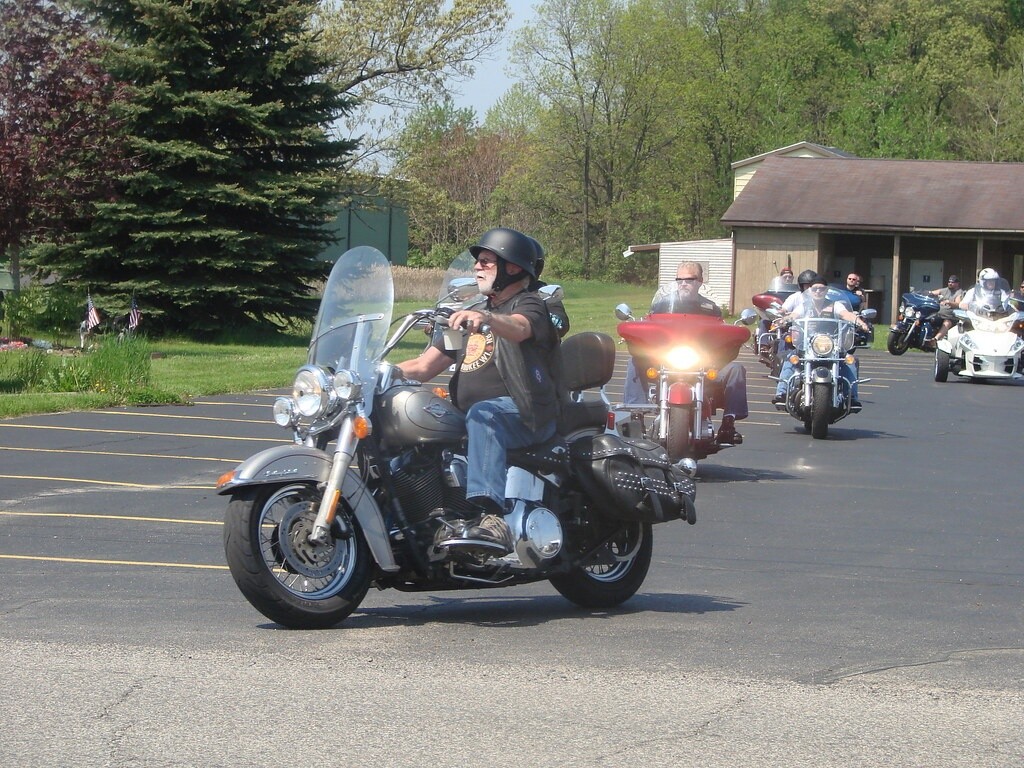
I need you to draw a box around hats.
[809,278,827,287]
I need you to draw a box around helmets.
[529,238,545,278]
[797,270,817,289]
[780,267,793,276]
[468,227,536,278]
[978,267,1000,288]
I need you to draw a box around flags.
[128,293,140,332]
[87,294,101,331]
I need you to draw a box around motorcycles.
[750,275,878,439]
[615,278,757,465]
[887,287,954,356]
[215,244,700,629]
[934,282,1024,383]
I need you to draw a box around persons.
[757,268,871,412]
[623,260,748,444]
[388,229,571,553]
[922,267,1024,349]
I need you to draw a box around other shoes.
[717,416,742,445]
[852,400,863,416]
[772,395,784,410]
[929,337,938,350]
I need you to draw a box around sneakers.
[468,514,515,553]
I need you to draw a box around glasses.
[848,278,857,281]
[675,278,697,284]
[474,258,498,269]
[811,286,827,291]
[1020,286,1024,289]
